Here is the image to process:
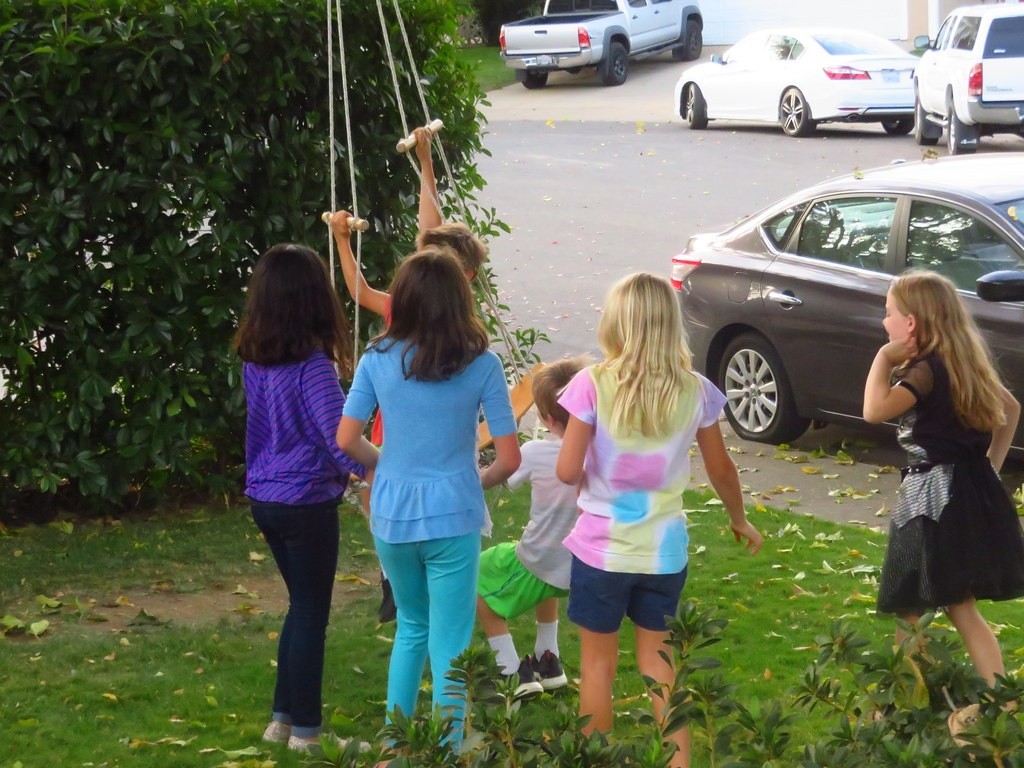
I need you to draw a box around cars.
[669,152,1023,463]
[674,26,922,137]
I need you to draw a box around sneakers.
[530,650,568,690]
[495,654,544,704]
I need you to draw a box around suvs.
[912,5,1024,156]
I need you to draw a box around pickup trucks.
[499,0,703,89]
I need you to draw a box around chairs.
[930,218,990,291]
[771,45,793,60]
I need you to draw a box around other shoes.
[264,722,292,741]
[288,731,372,754]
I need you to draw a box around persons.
[558,273,763,768]
[863,273,1023,715]
[234,243,373,755]
[331,126,486,624]
[475,359,591,702]
[336,249,521,768]
